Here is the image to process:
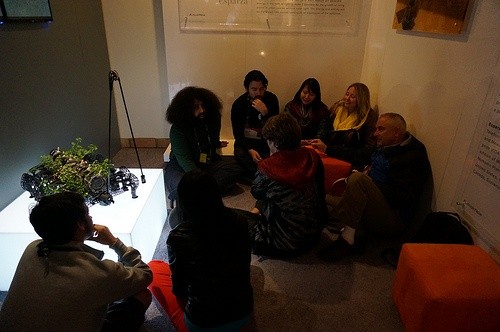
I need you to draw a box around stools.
[391,243,500,332]
[321,157,350,193]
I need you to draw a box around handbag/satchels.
[426,211,475,245]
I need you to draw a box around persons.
[164,87,236,215]
[326,113,434,262]
[0,190,154,332]
[282,78,330,150]
[250,111,328,260]
[327,83,378,171]
[147,168,254,332]
[231,69,279,185]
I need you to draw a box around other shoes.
[323,245,345,262]
